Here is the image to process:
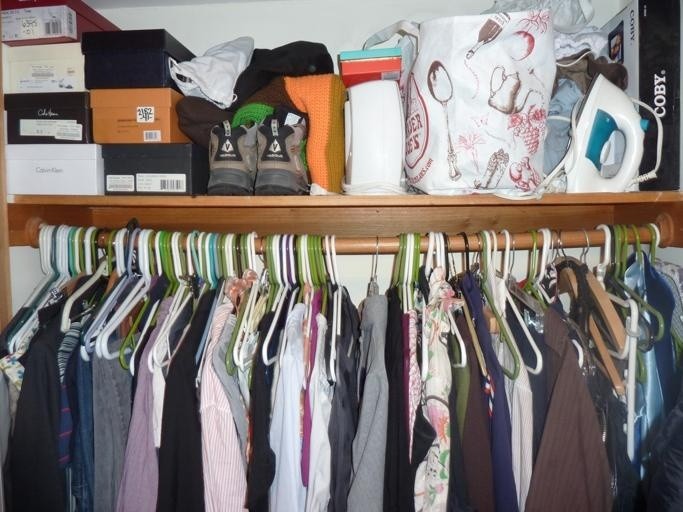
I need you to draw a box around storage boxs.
[2,41,87,94]
[2,145,106,196]
[2,91,92,144]
[101,144,210,195]
[80,28,200,96]
[1,1,122,46]
[89,88,196,145]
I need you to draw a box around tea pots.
[488,64,533,114]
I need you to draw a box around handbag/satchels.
[403,8,556,198]
[168,36,254,110]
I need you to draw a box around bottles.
[465,13,510,59]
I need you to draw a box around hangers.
[5,221,681,380]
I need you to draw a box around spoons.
[427,61,461,180]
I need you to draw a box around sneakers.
[204,107,307,196]
